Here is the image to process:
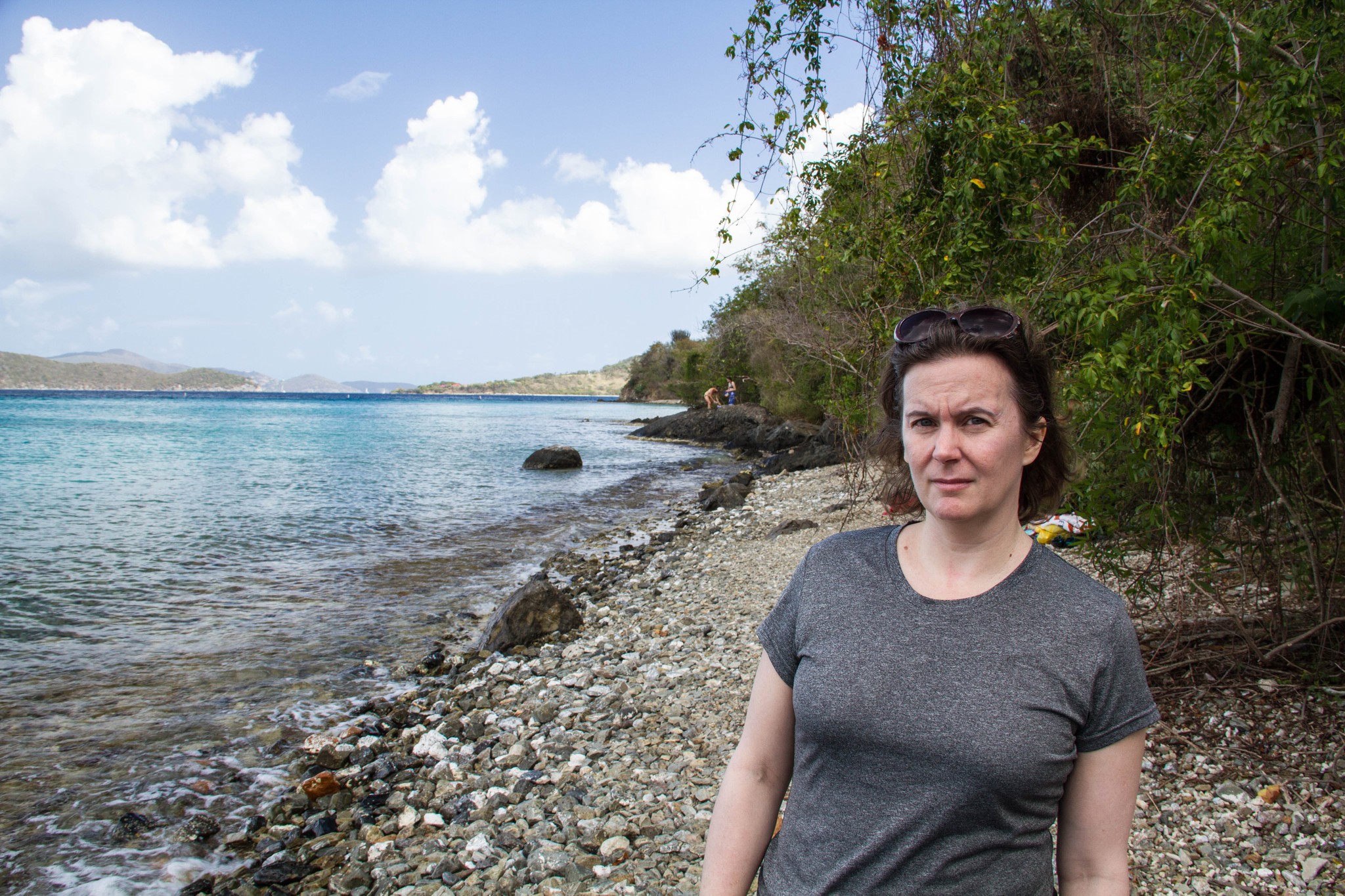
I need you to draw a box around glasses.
[892,306,1032,360]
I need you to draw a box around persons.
[704,385,722,409]
[696,306,1163,896]
[725,376,736,406]
